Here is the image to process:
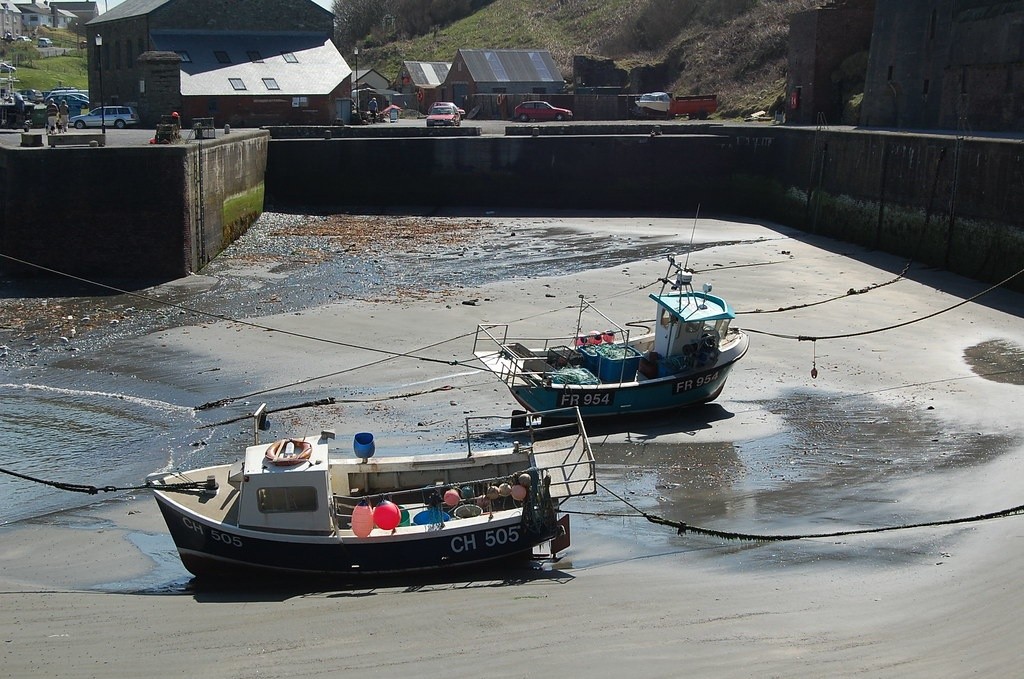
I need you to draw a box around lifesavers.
[264,437,312,467]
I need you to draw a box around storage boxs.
[577,343,620,375]
[548,345,573,367]
[595,346,644,382]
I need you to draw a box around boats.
[149,395,600,582]
[470,202,750,426]
[635,90,673,116]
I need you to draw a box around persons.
[14,93,24,113]
[59,100,69,132]
[45,98,59,134]
[369,97,378,123]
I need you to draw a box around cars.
[27,86,89,120]
[425,101,466,126]
[70,105,140,128]
[16,36,32,43]
[514,100,574,123]
[37,37,52,47]
[0,62,16,73]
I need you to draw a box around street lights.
[97,34,106,134]
[353,46,360,114]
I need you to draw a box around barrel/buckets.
[413,510,451,525]
[397,506,410,527]
[454,504,483,520]
[354,432,375,458]
